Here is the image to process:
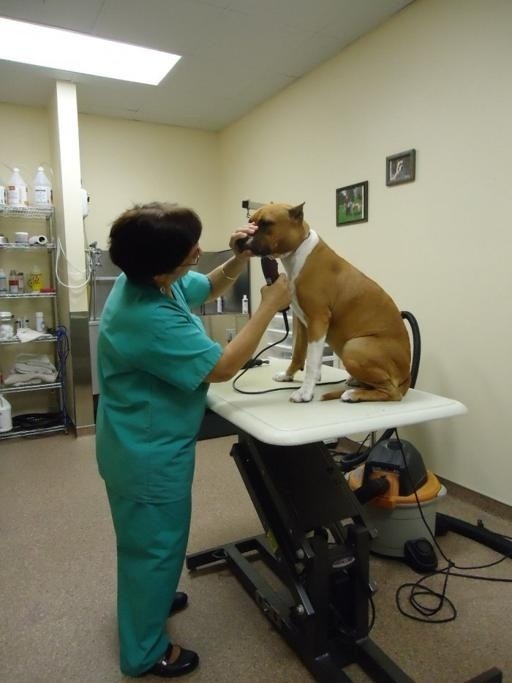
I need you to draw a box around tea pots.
[0,311,22,342]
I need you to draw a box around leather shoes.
[170,590,188,615]
[135,640,201,677]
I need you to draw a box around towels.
[2,352,59,386]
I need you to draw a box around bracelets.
[220,262,241,282]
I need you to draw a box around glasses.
[179,242,202,270]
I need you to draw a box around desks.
[185,357,502,681]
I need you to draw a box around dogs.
[232,201,413,404]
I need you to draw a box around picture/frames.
[335,180,369,226]
[385,148,417,187]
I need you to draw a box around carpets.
[93,393,240,440]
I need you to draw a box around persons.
[89,201,294,679]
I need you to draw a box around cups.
[15,232,28,243]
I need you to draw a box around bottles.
[17,311,46,333]
[0,392,13,433]
[242,294,248,315]
[29,165,53,210]
[0,232,9,246]
[217,295,222,313]
[0,264,47,295]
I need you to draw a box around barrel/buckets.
[32,167,52,206]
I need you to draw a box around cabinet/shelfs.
[1,204,68,439]
[265,304,340,368]
[198,313,252,349]
